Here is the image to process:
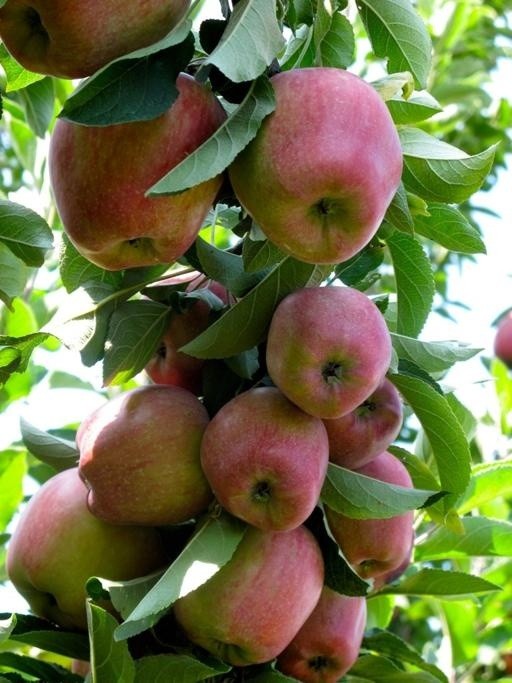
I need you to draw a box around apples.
[0,0,192,79]
[48,68,232,271]
[7,273,416,683]
[226,66,402,265]
[495,311,512,362]
